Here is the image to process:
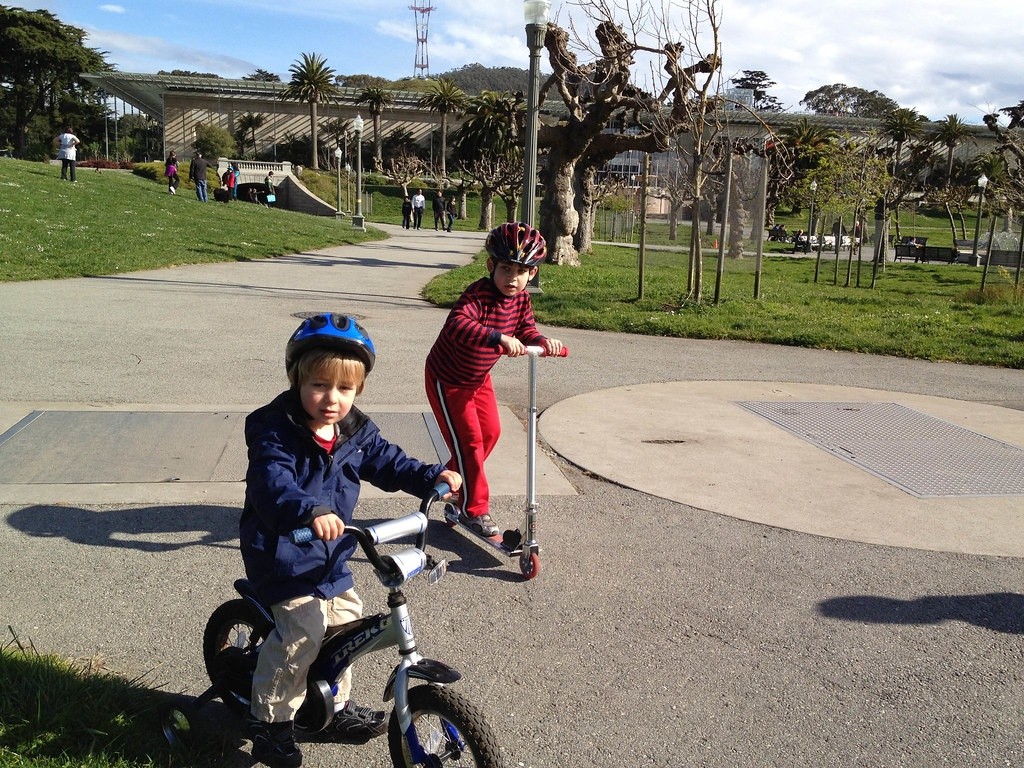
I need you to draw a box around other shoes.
[403,225,404,228]
[436,229,438,231]
[406,227,409,229]
[443,228,447,231]
[169,186,176,194]
[447,229,451,232]
[417,227,420,230]
[414,226,415,229]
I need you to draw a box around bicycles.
[202,483,505,768]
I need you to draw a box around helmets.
[285,314,376,377]
[486,222,546,267]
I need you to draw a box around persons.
[831,216,848,254]
[444,196,456,232]
[189,151,212,202]
[238,313,462,768]
[53,128,80,182]
[855,221,861,238]
[264,171,275,209]
[432,190,446,231]
[225,162,240,201]
[165,151,180,195]
[907,236,923,251]
[771,223,788,243]
[402,188,425,230]
[425,222,563,536]
[247,188,264,205]
[795,229,826,252]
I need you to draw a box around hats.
[227,167,233,170]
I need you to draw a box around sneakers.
[458,512,499,537]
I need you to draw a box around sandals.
[315,699,389,740]
[239,717,303,768]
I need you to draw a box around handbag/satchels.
[267,194,276,202]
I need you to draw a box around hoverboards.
[445,346,569,578]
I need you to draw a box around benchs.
[764,228,788,243]
[789,230,861,255]
[893,236,959,266]
[868,233,894,246]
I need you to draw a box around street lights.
[335,146,345,216]
[631,174,635,210]
[521,0,551,229]
[969,173,989,263]
[351,111,365,231]
[345,164,351,216]
[805,180,817,252]
